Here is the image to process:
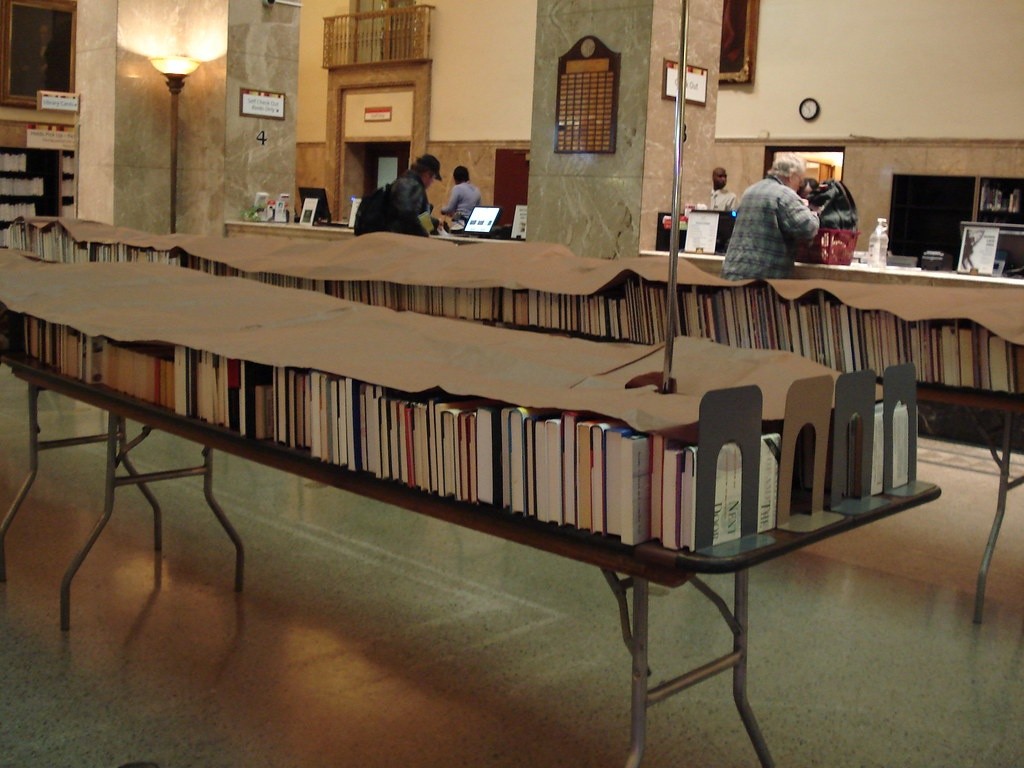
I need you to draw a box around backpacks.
[353,181,396,236]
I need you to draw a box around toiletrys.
[868,216,890,270]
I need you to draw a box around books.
[10,224,1024,551]
[0,154,75,222]
[981,185,1020,213]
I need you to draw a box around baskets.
[794,228,861,265]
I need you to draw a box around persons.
[387,154,443,236]
[720,156,820,281]
[799,177,824,213]
[710,168,738,211]
[442,166,481,221]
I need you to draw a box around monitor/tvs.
[463,206,502,235]
[960,221,1024,279]
[692,210,737,253]
[298,187,332,225]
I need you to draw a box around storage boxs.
[791,230,863,264]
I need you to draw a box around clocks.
[799,98,819,121]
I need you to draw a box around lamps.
[148,53,201,232]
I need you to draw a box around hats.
[417,153,442,181]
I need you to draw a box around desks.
[0,350,941,767]
[906,380,1024,624]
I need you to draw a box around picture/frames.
[719,0,760,84]
[0,0,77,108]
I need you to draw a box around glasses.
[794,171,803,186]
[718,174,727,177]
